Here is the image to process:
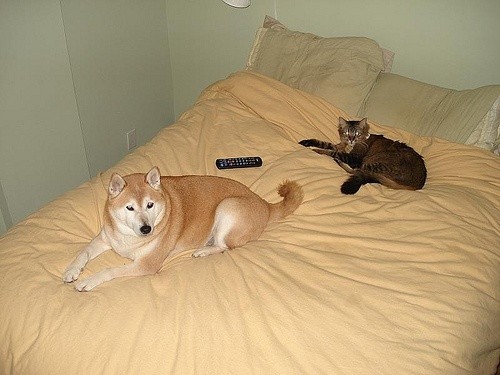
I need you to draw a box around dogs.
[61,166,304,292]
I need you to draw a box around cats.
[298,115,427,197]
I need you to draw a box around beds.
[1,15,500,375]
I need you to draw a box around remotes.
[216,157,262,169]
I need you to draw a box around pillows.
[246,15,393,117]
[360,73,499,156]
